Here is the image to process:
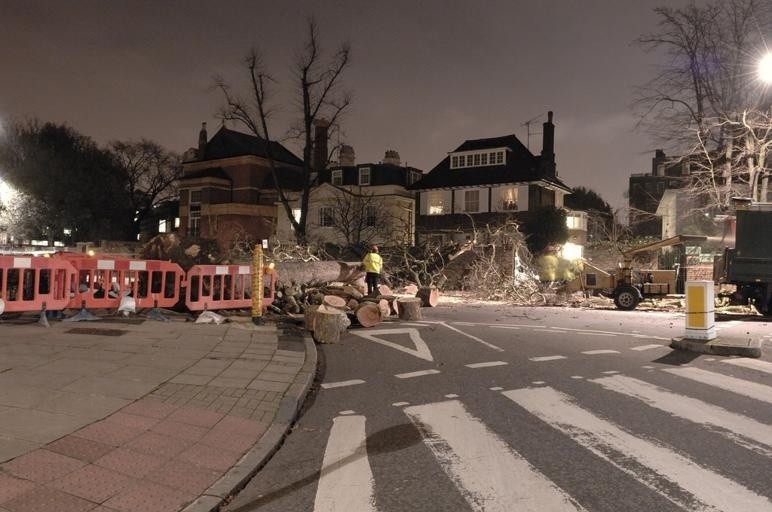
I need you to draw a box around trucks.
[713,202,772,319]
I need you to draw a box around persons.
[361,242,384,297]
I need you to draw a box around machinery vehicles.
[574,235,708,311]
